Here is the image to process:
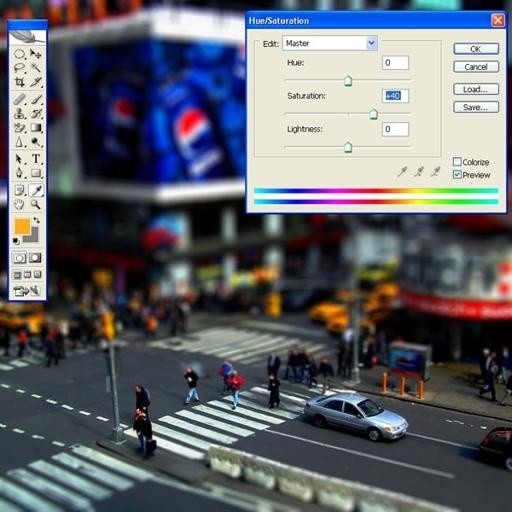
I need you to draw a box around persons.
[267,372,281,410]
[0,295,186,369]
[266,351,281,390]
[135,383,150,423]
[226,368,244,410]
[218,358,234,397]
[478,346,511,406]
[180,366,200,406]
[281,334,373,387]
[134,406,152,460]
